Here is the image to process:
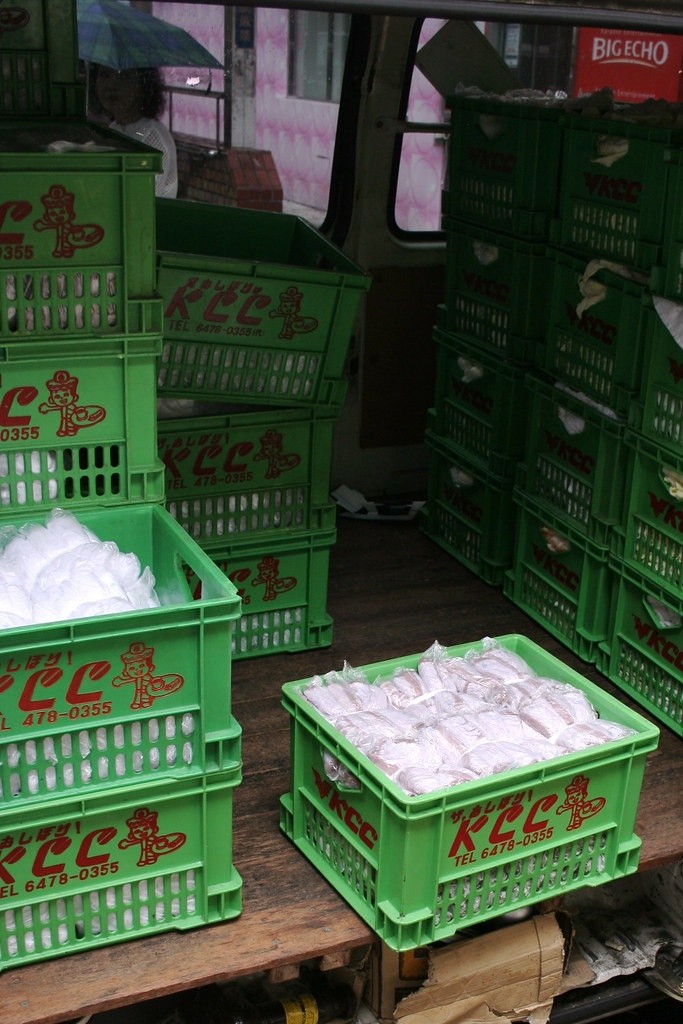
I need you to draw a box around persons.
[89,65,177,200]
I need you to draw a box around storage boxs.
[419,431,516,587]
[0,763,244,972]
[153,196,371,412]
[441,93,581,239]
[206,533,337,661]
[512,370,627,547]
[627,144,683,456]
[546,113,683,275]
[435,215,551,367]
[0,335,164,519]
[279,633,660,953]
[0,0,85,122]
[595,553,683,737]
[425,325,530,484]
[502,488,610,665]
[0,503,243,810]
[609,428,683,601]
[0,121,163,346]
[158,409,337,551]
[533,244,647,418]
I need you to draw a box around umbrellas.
[75,0,226,70]
